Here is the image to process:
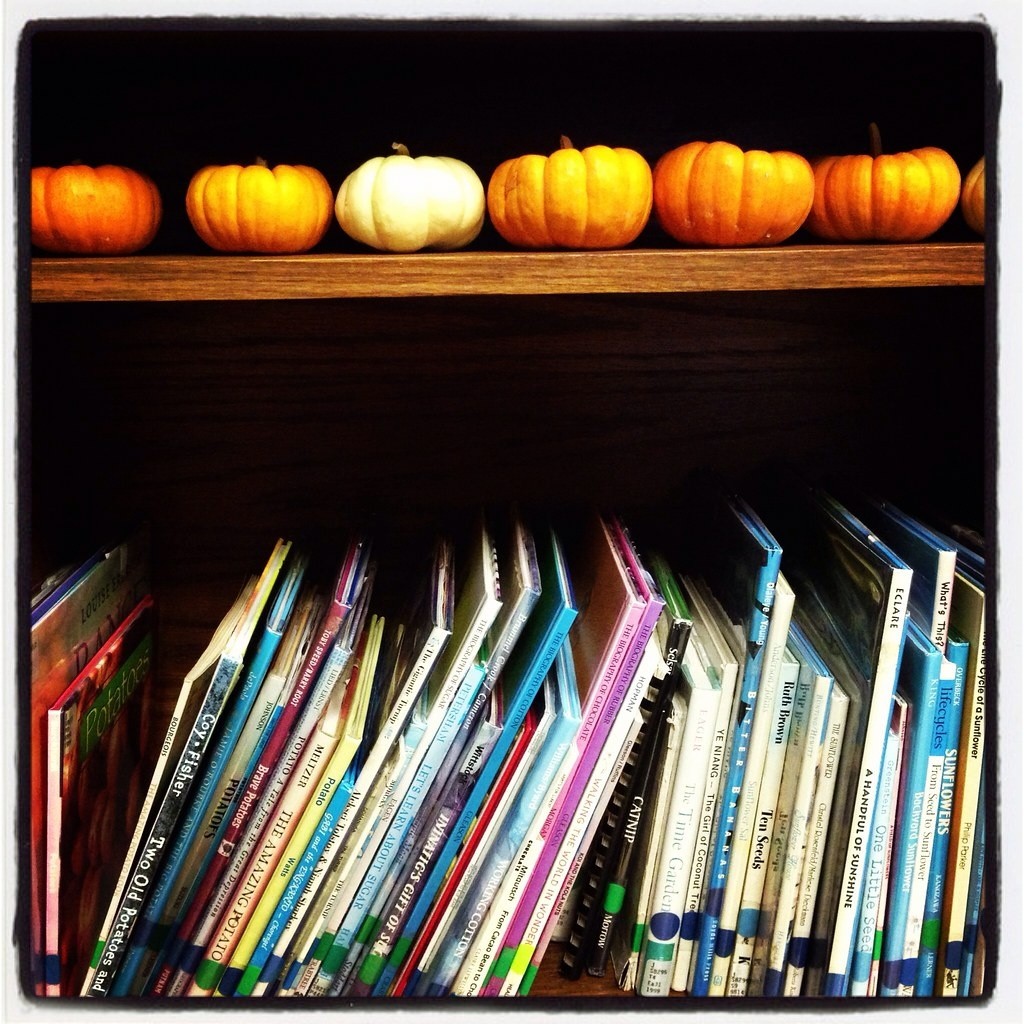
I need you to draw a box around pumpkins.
[183,139,987,253]
[30,163,159,256]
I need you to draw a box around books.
[30,497,993,1001]
